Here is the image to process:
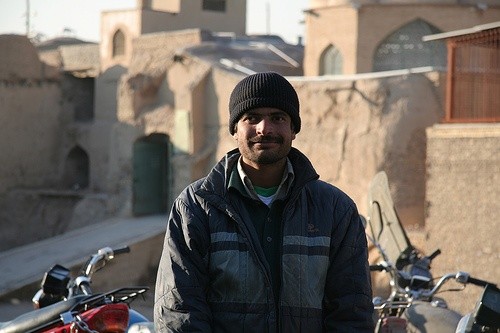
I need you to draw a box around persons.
[154,72,374,333]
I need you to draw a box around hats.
[229,72,301,136]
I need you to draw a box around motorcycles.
[32,244,154,333]
[359,171,500,333]
[0,286,150,333]
